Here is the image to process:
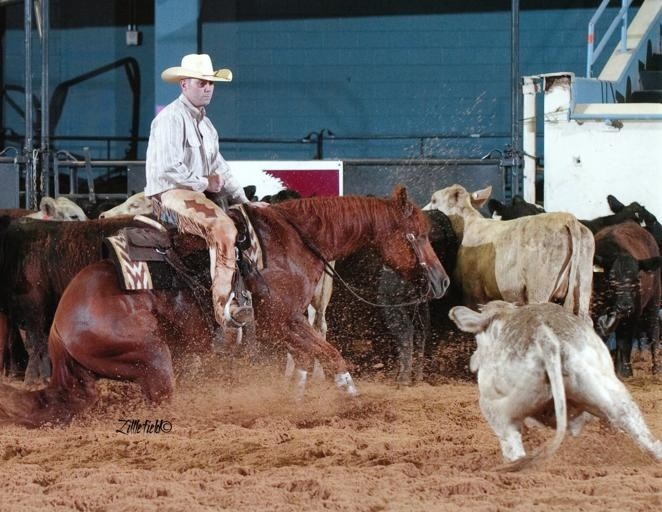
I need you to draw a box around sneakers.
[223,306,254,328]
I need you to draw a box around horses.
[0,185,451,431]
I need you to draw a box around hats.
[161,53,233,83]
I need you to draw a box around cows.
[486,192,662,380]
[0,185,462,392]
[448,299,662,473]
[421,182,597,326]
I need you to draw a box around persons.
[145,54,269,329]
